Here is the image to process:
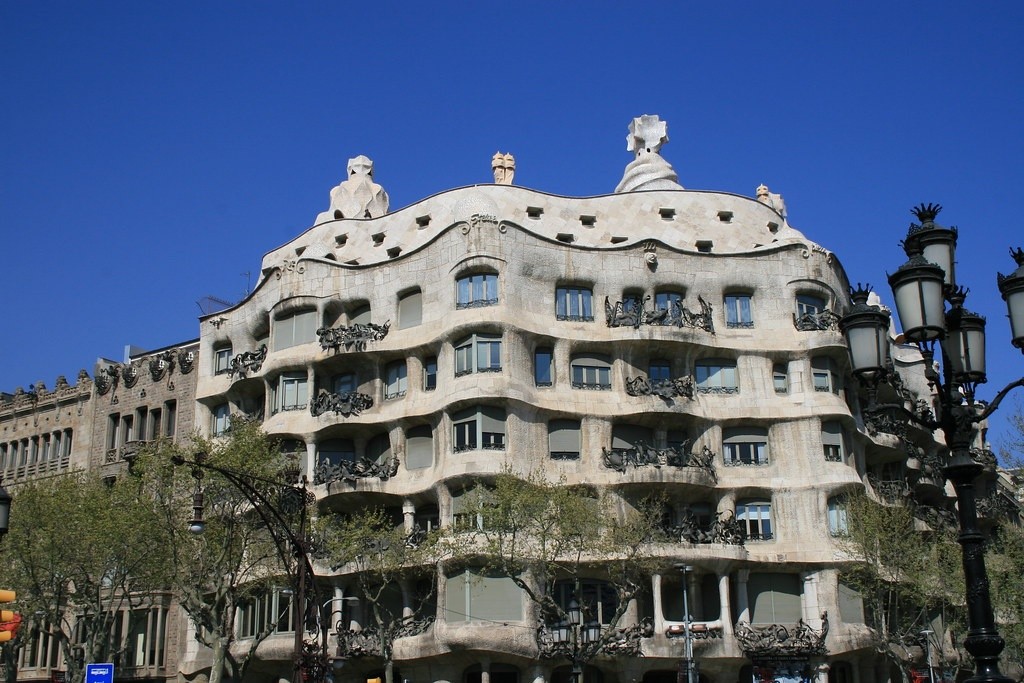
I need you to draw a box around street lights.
[839,201,1024,683]
[552,597,602,683]
[171,453,329,683]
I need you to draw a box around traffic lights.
[1,589,16,643]
